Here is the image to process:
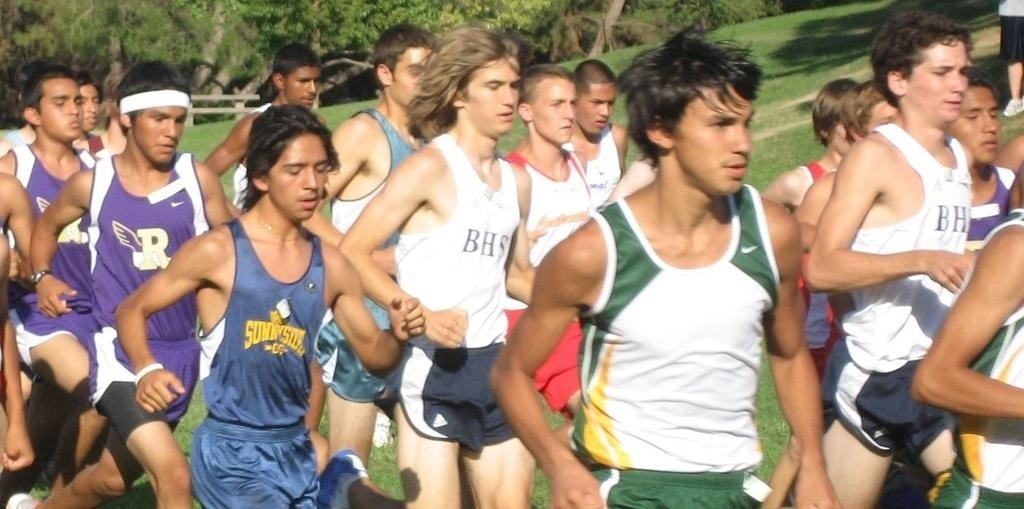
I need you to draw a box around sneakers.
[315,448,369,509]
[5,492,38,509]
[1002,95,1024,117]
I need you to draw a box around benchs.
[181,93,263,126]
